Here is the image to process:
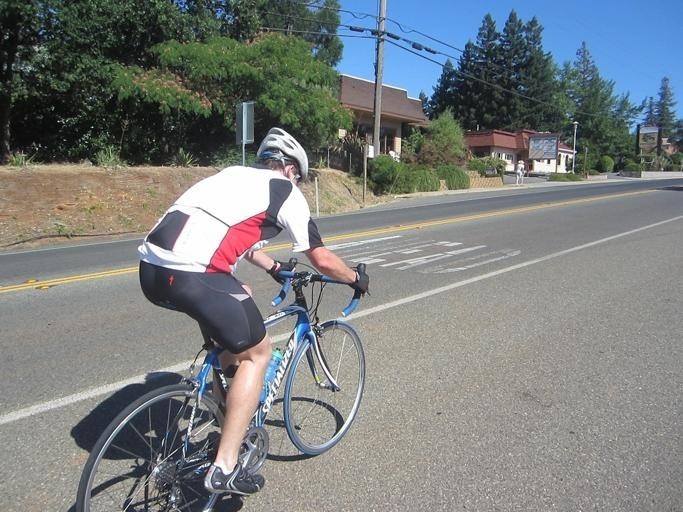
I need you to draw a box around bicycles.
[76,258,371,512]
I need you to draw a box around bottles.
[258,347,283,405]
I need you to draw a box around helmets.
[256,126,309,183]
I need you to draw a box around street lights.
[572,120,579,174]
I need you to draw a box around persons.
[515,159,525,186]
[138,125,371,497]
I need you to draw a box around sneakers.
[203,459,265,496]
